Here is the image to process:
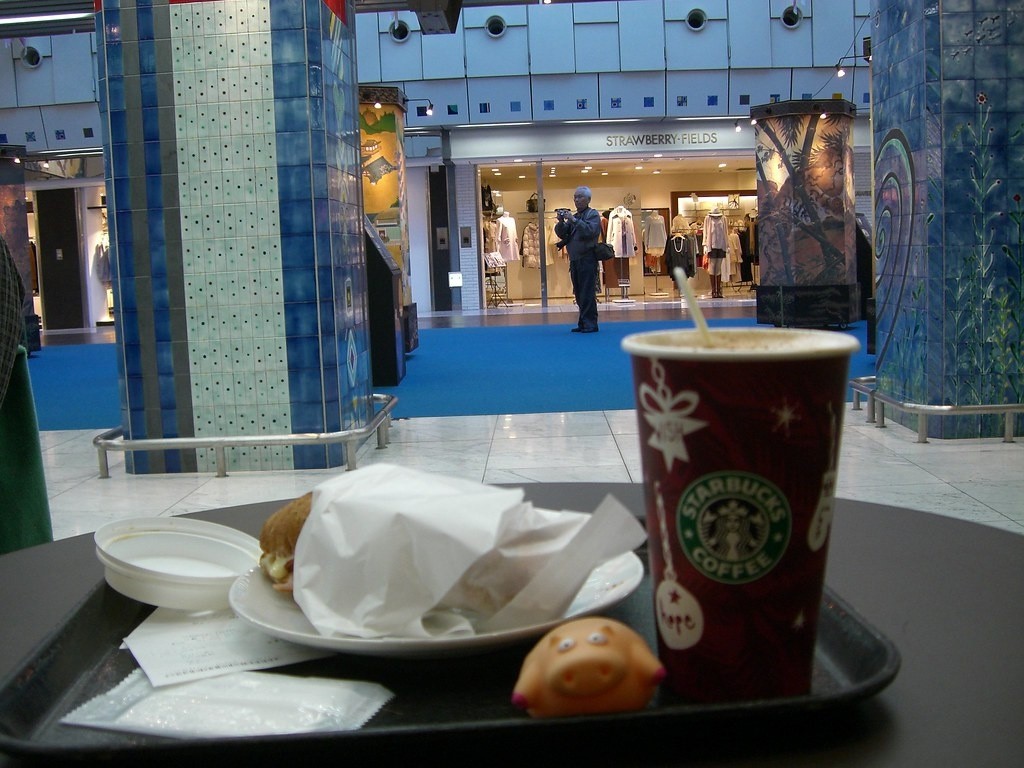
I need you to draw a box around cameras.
[558,209,567,218]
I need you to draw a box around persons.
[702,207,728,297]
[554,186,601,333]
[645,210,667,253]
[673,213,690,230]
[495,211,520,262]
[607,205,637,258]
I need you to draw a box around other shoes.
[572,326,598,333]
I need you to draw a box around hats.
[708,207,722,216]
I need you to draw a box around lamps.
[835,53,873,78]
[735,118,743,132]
[403,97,434,115]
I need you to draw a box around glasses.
[573,195,585,199]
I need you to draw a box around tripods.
[485,251,509,309]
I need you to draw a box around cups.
[624,327,859,697]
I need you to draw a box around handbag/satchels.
[527,193,545,212]
[594,242,614,260]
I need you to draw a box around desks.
[0,482,1024,768]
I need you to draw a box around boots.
[710,274,724,298]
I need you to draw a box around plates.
[231,545,642,650]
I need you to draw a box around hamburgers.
[259,490,313,592]
